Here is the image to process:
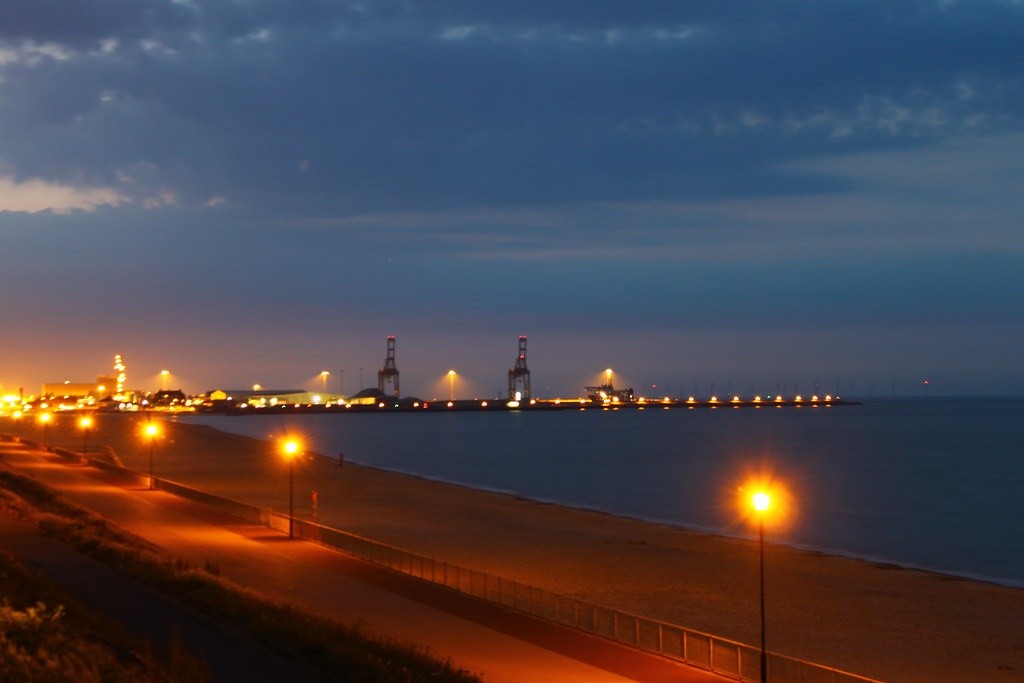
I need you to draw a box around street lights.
[40,413,51,446]
[79,415,93,455]
[141,421,160,491]
[736,473,796,683]
[274,428,306,538]
